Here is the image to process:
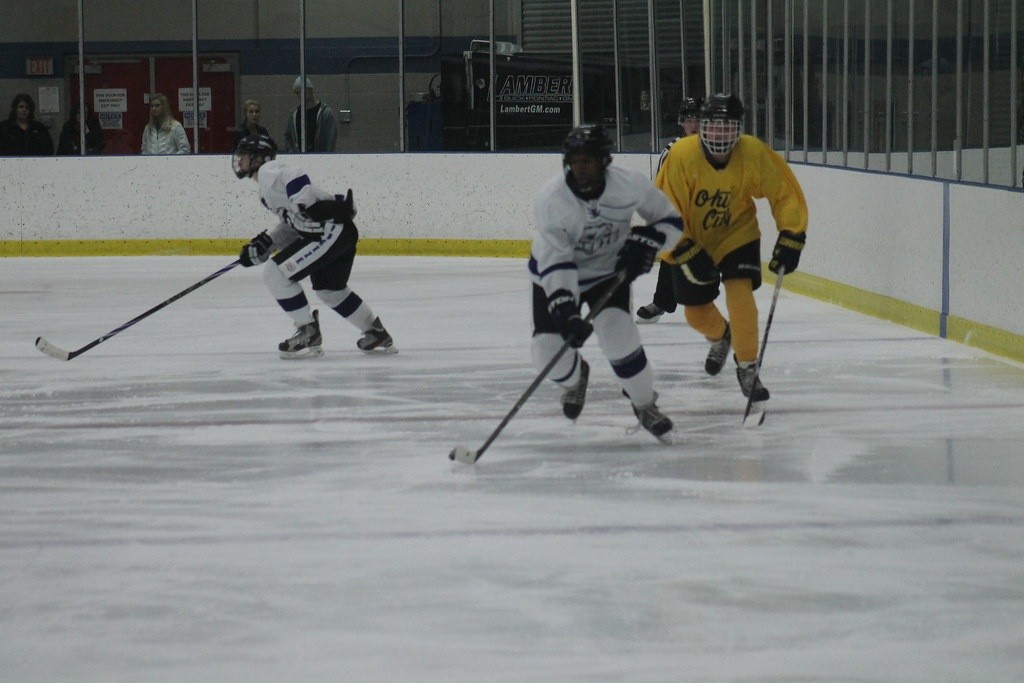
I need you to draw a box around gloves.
[672,241,717,285]
[547,288,593,347]
[614,227,665,279]
[285,210,324,242]
[769,232,805,275]
[240,232,276,267]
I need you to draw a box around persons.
[60,103,106,156]
[0,94,54,157]
[649,92,808,412]
[230,99,269,151]
[525,125,684,448]
[636,100,702,326]
[285,77,337,153]
[140,94,192,155]
[231,134,400,361]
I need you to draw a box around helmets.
[561,124,614,187]
[232,134,277,179]
[677,98,700,121]
[700,93,744,155]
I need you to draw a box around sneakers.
[560,363,589,425]
[635,303,664,325]
[634,404,673,446]
[705,327,730,376]
[734,365,769,408]
[280,322,326,359]
[357,332,399,356]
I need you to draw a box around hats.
[293,76,312,89]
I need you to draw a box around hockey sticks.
[34,256,246,362]
[449,269,626,466]
[741,267,786,425]
